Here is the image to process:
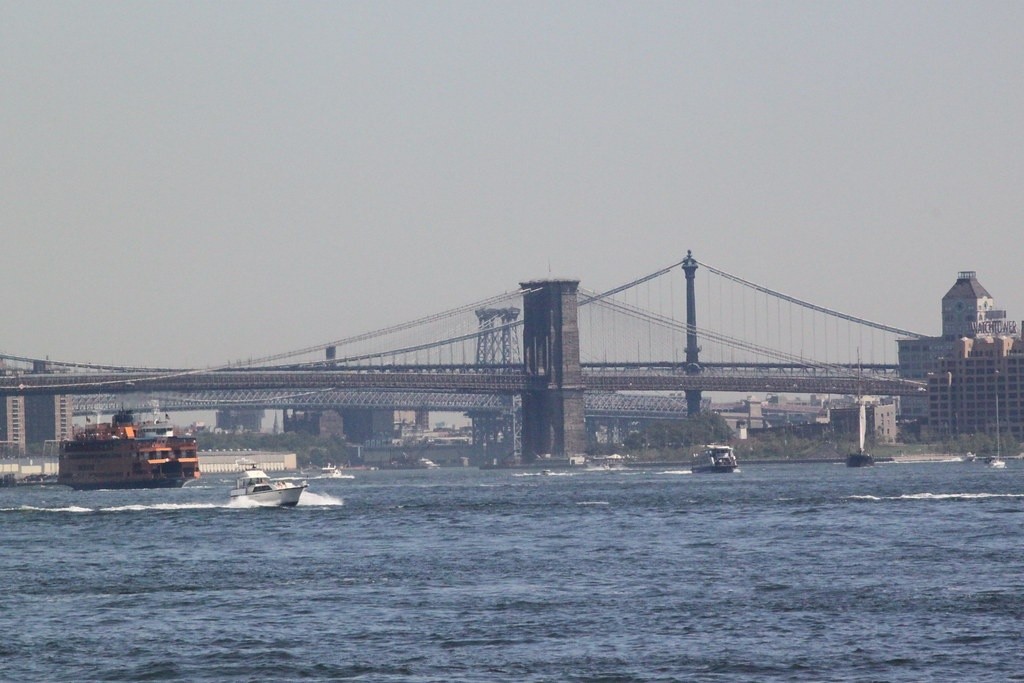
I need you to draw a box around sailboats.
[983,369,1008,470]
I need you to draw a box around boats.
[319,463,343,479]
[390,452,441,469]
[690,443,739,473]
[56,377,202,489]
[844,448,875,467]
[228,462,309,507]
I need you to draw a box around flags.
[86,415,90,423]
[165,413,170,420]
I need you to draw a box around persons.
[255,478,259,484]
[248,478,255,485]
